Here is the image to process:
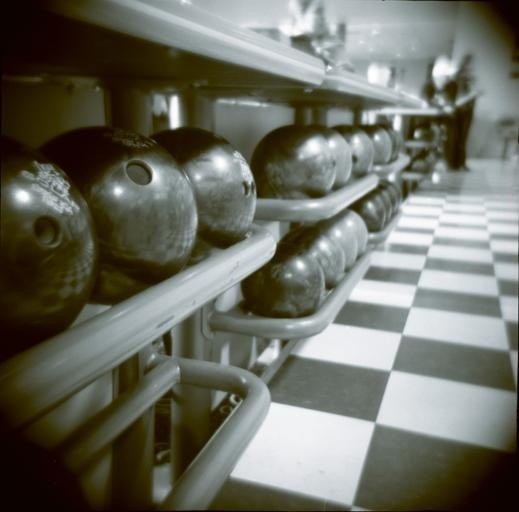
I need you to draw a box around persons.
[440,54,479,173]
